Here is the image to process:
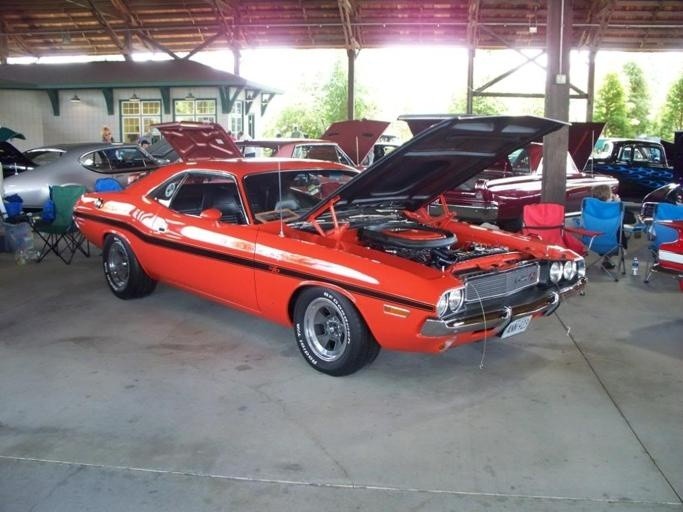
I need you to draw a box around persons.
[227,124,311,158]
[127,124,161,149]
[102,127,117,161]
[589,183,635,270]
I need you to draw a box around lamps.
[185,86,195,101]
[129,87,139,102]
[70,89,80,102]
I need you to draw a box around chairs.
[202,192,245,224]
[95,178,123,191]
[520,197,683,284]
[266,183,299,210]
[14,185,90,264]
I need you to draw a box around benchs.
[167,183,258,214]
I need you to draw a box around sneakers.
[601,262,615,269]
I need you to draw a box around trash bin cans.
[2,215,33,253]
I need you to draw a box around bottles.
[631,256,640,276]
